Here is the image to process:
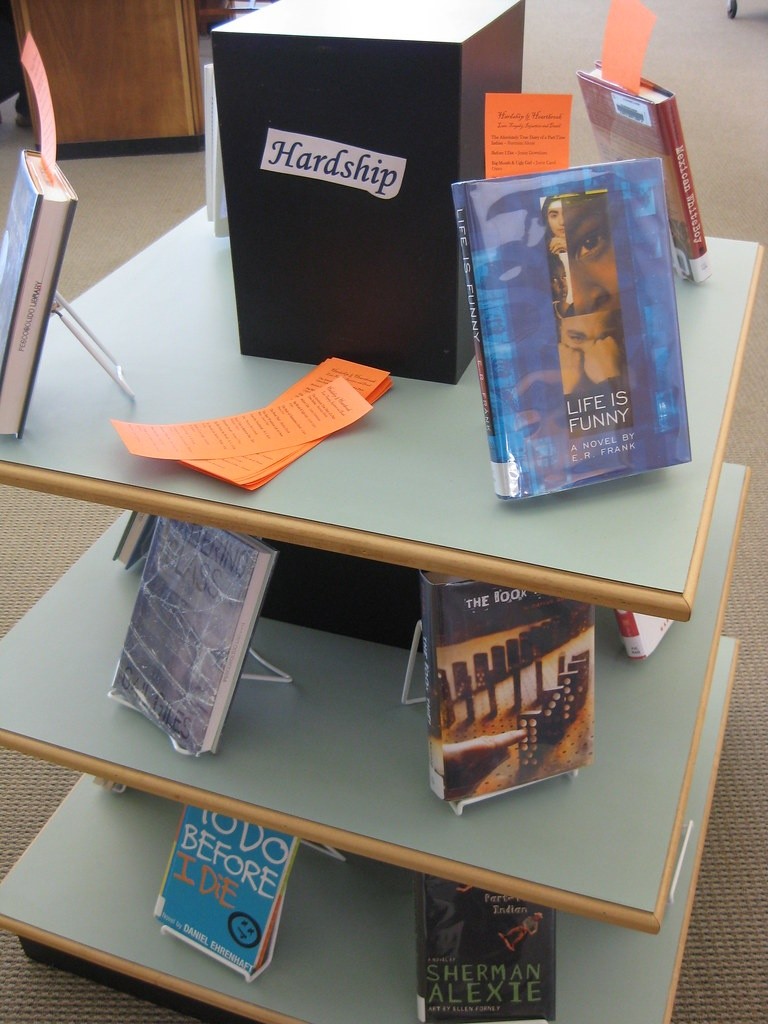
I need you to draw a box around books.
[1,63,716,1024]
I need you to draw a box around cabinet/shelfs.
[0,205,768,1024]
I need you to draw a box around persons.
[547,254,574,315]
[541,197,567,253]
[562,190,620,316]
[559,311,628,432]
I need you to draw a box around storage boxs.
[211,0,525,382]
